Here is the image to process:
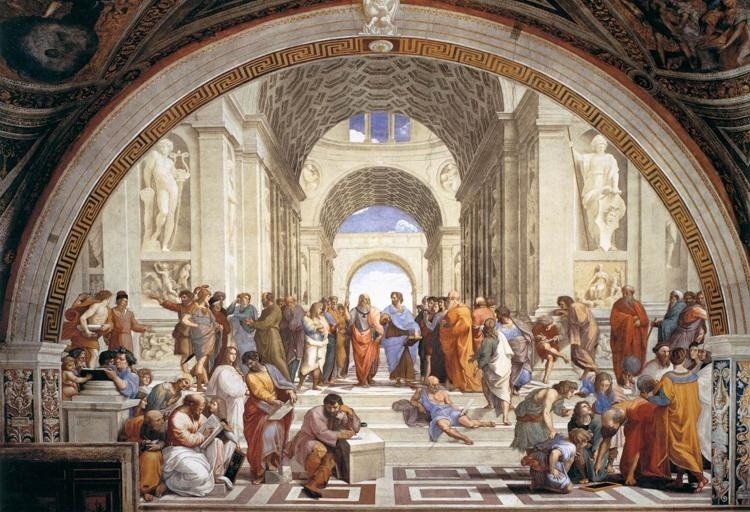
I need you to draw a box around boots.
[304,461,330,497]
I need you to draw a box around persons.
[567,133,628,251]
[300,257,307,302]
[454,254,461,292]
[297,163,320,192]
[634,0,750,74]
[61,278,713,500]
[440,163,460,195]
[130,138,191,253]
